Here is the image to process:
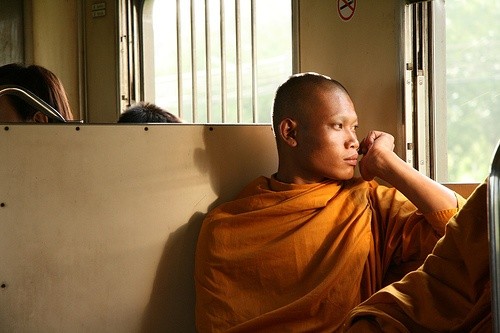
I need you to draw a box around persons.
[197,73,468,333]
[0,63,73,123]
[119,103,181,123]
[346,140,499,333]
